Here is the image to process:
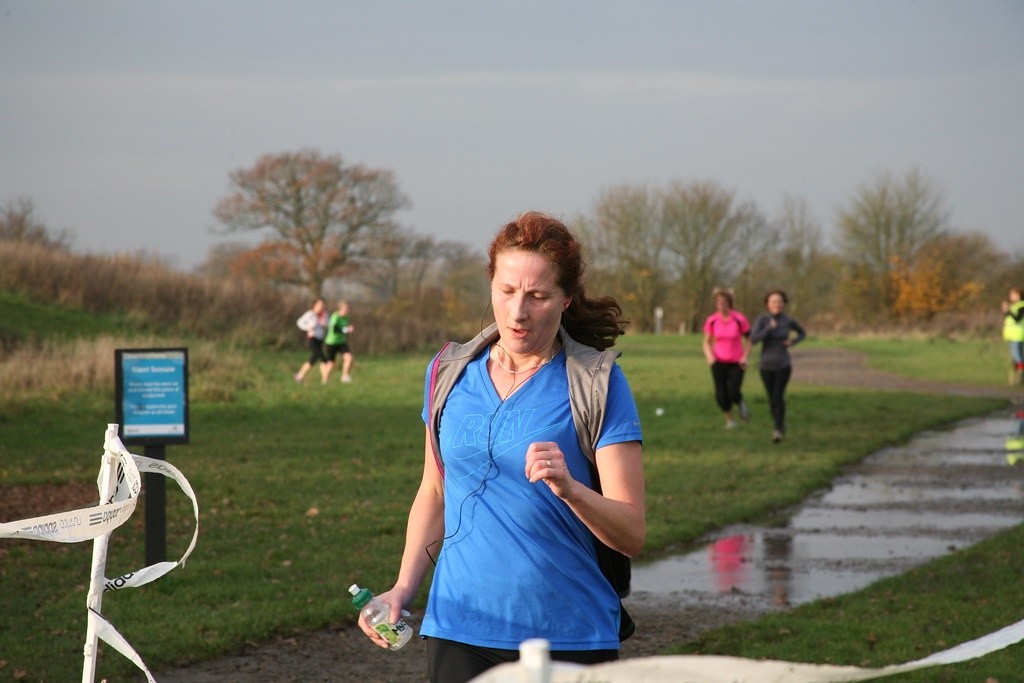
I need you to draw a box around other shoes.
[740,406,751,420]
[773,430,784,445]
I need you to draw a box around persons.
[702,285,752,431]
[1008,410,1024,464]
[752,290,807,444]
[322,299,354,384]
[359,210,647,683]
[294,297,330,384]
[1001,286,1024,383]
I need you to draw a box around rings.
[546,459,551,467]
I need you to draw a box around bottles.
[349,584,413,651]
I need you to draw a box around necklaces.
[495,334,557,374]
